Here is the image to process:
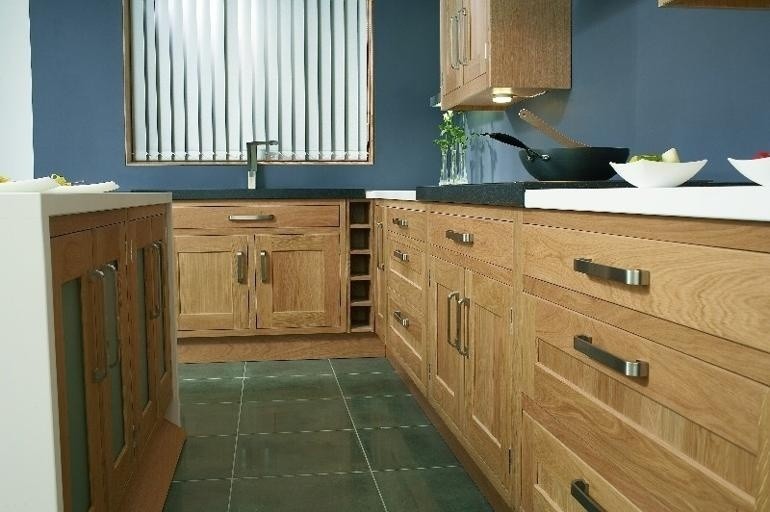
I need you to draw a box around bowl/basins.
[728,156,770,184]
[608,158,709,188]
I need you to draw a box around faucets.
[246,141,278,188]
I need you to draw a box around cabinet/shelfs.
[439,0,572,111]
[375,200,770,510]
[174,201,371,338]
[51,201,186,510]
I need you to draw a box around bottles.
[438,149,469,185]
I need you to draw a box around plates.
[46,181,120,193]
[0,177,58,193]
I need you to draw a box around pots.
[481,128,630,180]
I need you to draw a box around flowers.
[434,110,479,154]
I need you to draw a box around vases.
[439,143,468,186]
